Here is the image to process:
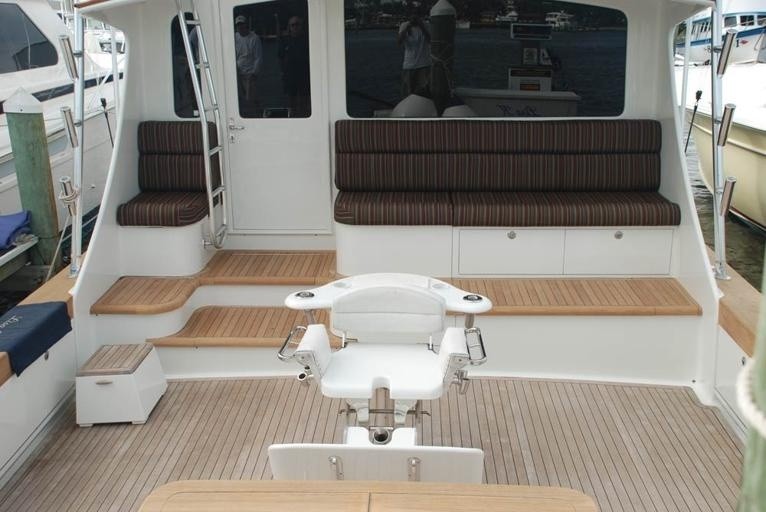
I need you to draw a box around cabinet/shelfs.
[335,226,677,281]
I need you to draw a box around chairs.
[267,444,485,487]
[116,120,227,278]
[279,270,493,444]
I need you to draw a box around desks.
[140,478,604,512]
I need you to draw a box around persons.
[181,24,201,115]
[388,0,480,117]
[230,0,377,118]
[396,0,431,103]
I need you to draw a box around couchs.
[329,119,682,226]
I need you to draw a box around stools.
[76,342,168,427]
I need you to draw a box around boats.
[344,0,592,35]
[674,0,765,64]
[680,104,765,236]
[0,0,127,287]
